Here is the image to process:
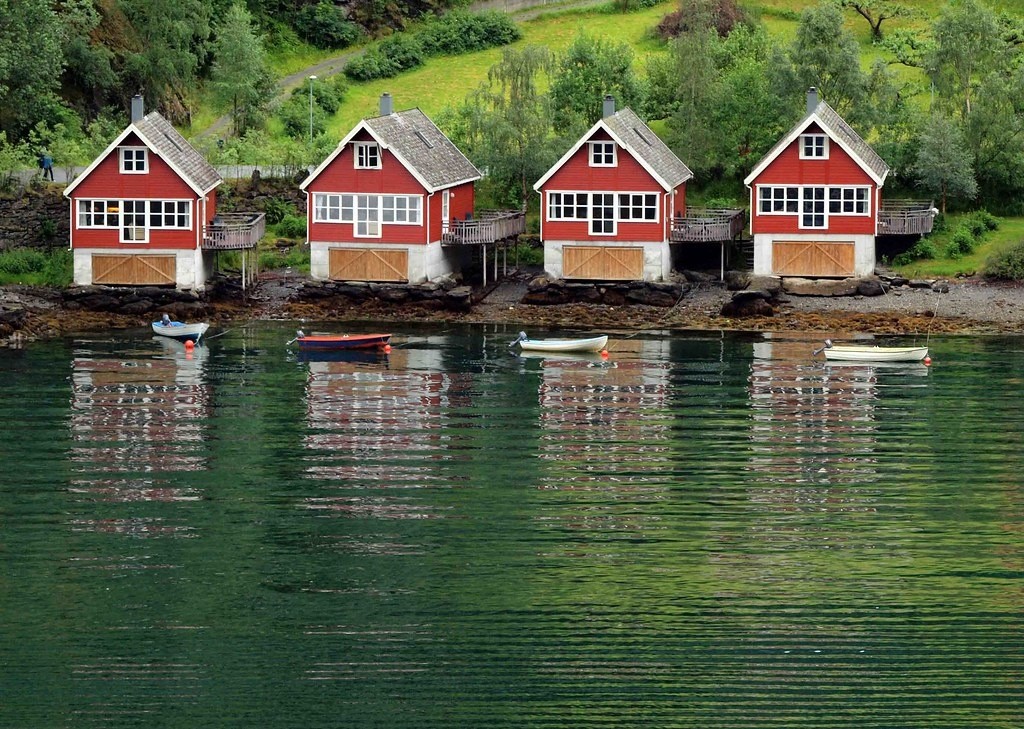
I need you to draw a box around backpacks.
[38,159,44,169]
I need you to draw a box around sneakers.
[51,180,56,183]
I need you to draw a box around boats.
[519,336,608,352]
[152,321,210,347]
[665,327,764,339]
[824,346,928,360]
[296,334,393,361]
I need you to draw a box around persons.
[43,150,56,182]
